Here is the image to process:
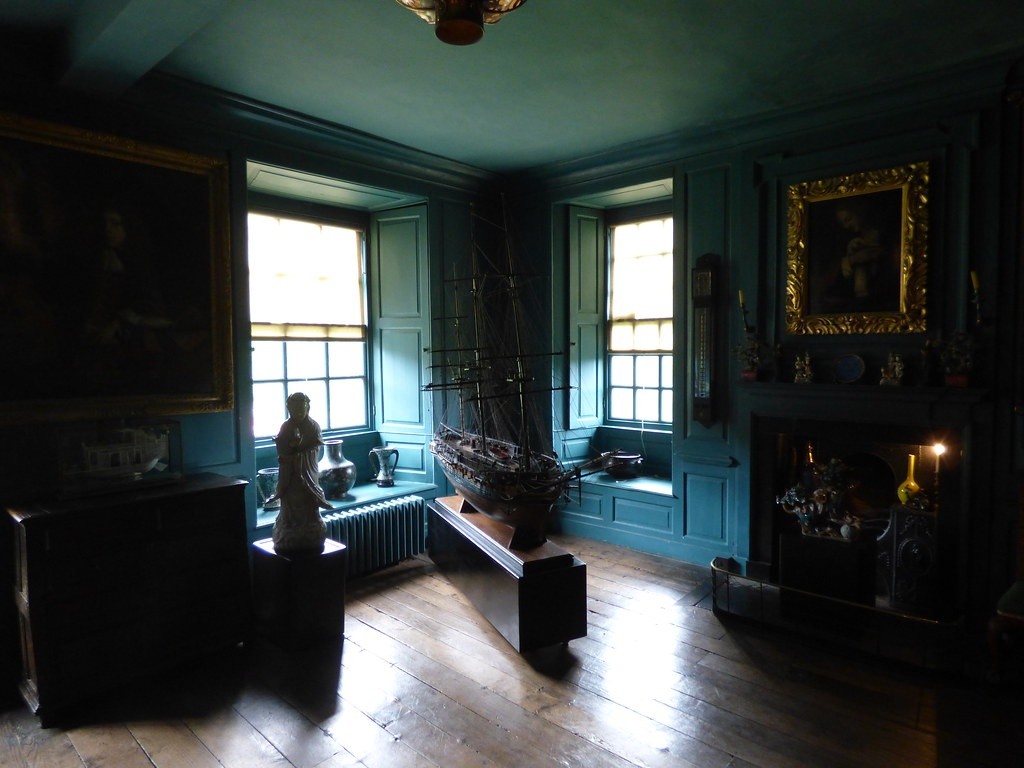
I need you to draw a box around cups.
[369,446,399,487]
[256,467,281,510]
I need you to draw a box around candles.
[971,271,980,289]
[739,290,743,304]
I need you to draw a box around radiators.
[321,494,425,578]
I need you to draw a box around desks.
[426,495,587,654]
[709,556,968,684]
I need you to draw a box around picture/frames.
[0,111,233,427]
[785,160,928,336]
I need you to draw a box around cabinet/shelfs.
[2,472,251,729]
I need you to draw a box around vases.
[317,440,357,500]
[601,450,643,477]
[898,454,920,504]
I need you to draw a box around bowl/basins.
[603,451,643,478]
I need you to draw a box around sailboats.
[420,197,628,551]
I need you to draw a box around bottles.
[897,454,920,504]
[318,439,356,499]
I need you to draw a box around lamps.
[396,0,527,46]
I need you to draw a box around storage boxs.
[33,420,184,503]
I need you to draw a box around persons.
[796,356,806,375]
[264,394,333,522]
[889,353,904,381]
